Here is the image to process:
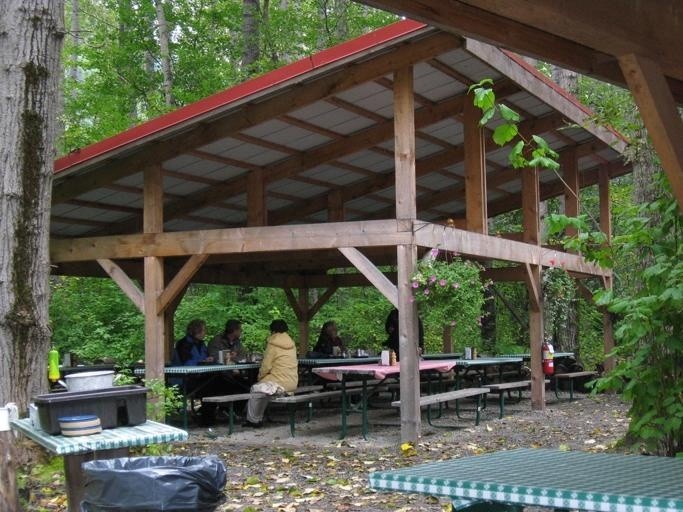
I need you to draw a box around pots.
[58,370,121,393]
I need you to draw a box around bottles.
[472,348,477,360]
[391,350,397,366]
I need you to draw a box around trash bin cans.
[81,455,219,512]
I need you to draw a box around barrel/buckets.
[63,370,115,393]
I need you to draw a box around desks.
[134,361,260,420]
[297,357,456,445]
[9,416,186,511]
[368,448,683,512]
[419,350,575,387]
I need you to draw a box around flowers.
[539,257,575,342]
[406,243,494,349]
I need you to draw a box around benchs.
[202,385,324,434]
[480,377,552,419]
[552,369,597,404]
[391,384,492,428]
[270,385,387,437]
[327,368,519,401]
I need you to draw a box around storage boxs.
[30,385,152,434]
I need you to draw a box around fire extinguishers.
[542,338,555,375]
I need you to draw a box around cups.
[7,402,19,421]
[0,408,12,432]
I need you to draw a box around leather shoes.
[239,419,263,429]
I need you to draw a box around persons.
[239,319,299,430]
[206,318,256,422]
[314,319,363,409]
[169,318,233,428]
[382,306,424,359]
